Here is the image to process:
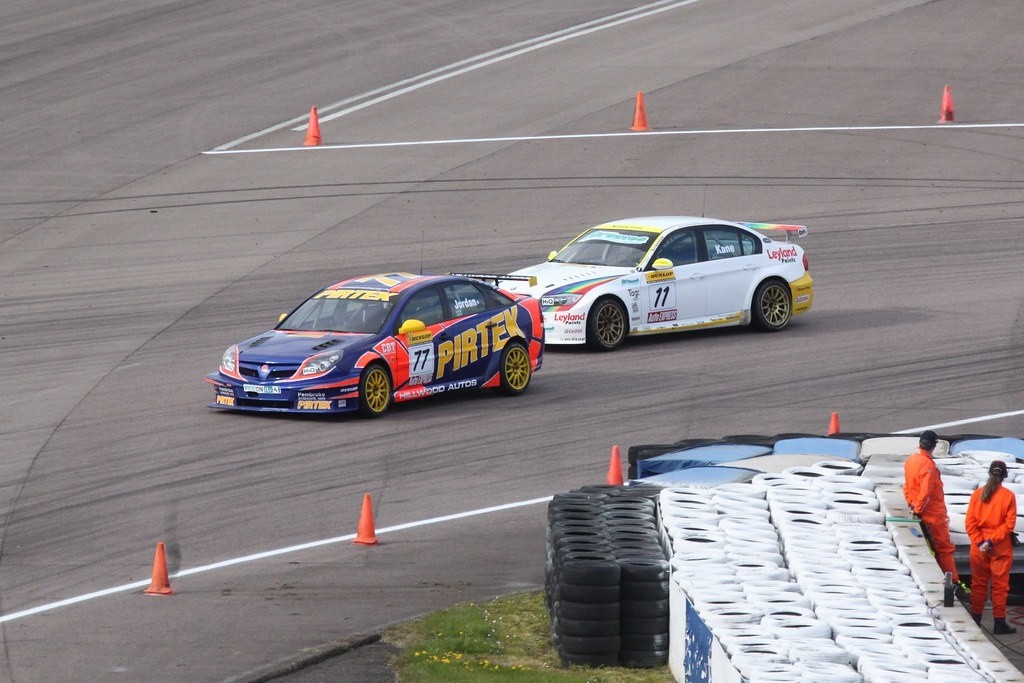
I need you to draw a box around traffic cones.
[629,92,654,131]
[143,542,177,595]
[606,445,624,486]
[938,84,958,124]
[827,413,840,437]
[351,492,378,544]
[301,105,324,146]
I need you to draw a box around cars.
[200,270,546,421]
[493,216,817,355]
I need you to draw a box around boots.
[972,613,982,630]
[992,616,1018,635]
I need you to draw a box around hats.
[918,430,939,444]
[988,460,1009,477]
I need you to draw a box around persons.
[903,428,972,599]
[963,460,1021,633]
